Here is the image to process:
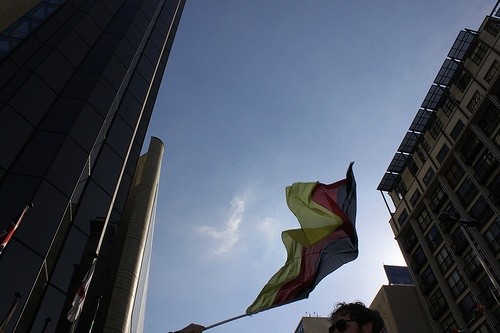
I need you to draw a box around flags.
[245,161,359,315]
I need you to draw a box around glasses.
[329,319,353,332]
